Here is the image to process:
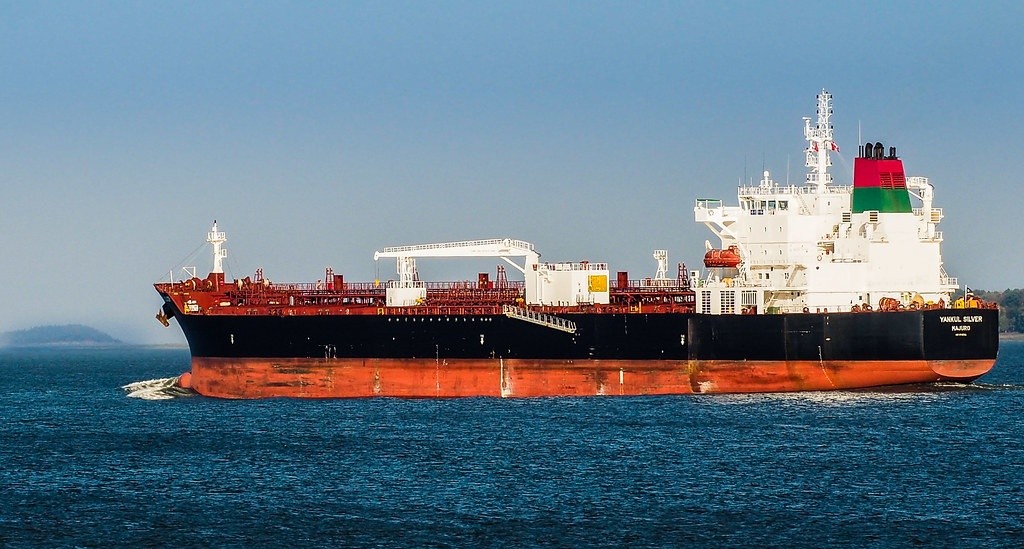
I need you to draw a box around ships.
[151,84,1002,401]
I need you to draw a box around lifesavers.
[708,210,714,216]
[817,255,822,261]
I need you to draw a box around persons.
[315,279,322,291]
